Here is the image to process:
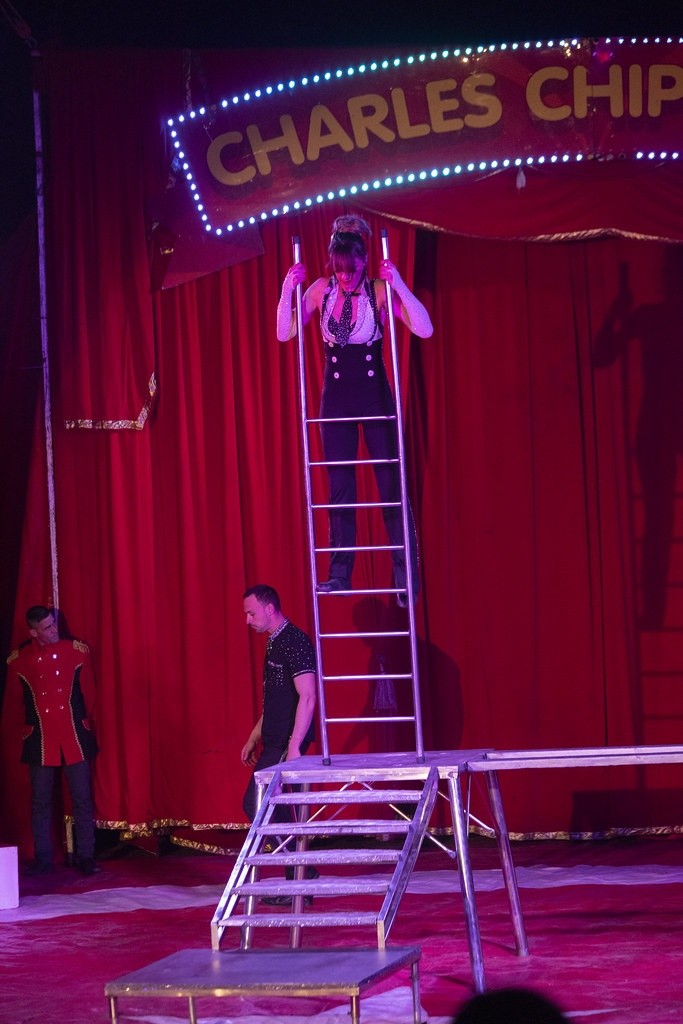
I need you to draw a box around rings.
[384,262,387,266]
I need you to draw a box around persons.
[275,217,435,604]
[6,605,106,875]
[449,984,570,1024]
[241,583,318,904]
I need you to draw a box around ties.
[335,268,366,347]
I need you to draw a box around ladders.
[291,228,426,768]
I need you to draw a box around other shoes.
[262,896,312,907]
[318,578,350,597]
[22,862,51,876]
[396,589,415,608]
[303,868,319,881]
[75,856,100,874]
